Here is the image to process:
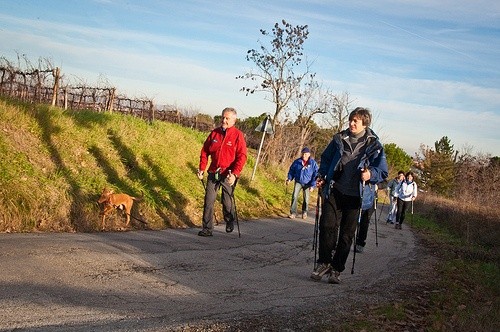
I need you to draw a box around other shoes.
[386,219,392,223]
[390,221,395,224]
[302,213,308,219]
[395,224,398,229]
[289,214,296,219]
[355,245,364,253]
[399,224,402,230]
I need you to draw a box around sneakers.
[226,221,234,232]
[312,263,330,281]
[198,228,214,237]
[329,266,341,284]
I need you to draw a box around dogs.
[98,188,144,229]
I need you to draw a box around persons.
[311,107,389,282]
[393,171,417,229]
[386,170,405,224]
[286,148,318,219]
[197,108,247,236]
[334,185,378,252]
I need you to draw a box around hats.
[301,148,310,154]
